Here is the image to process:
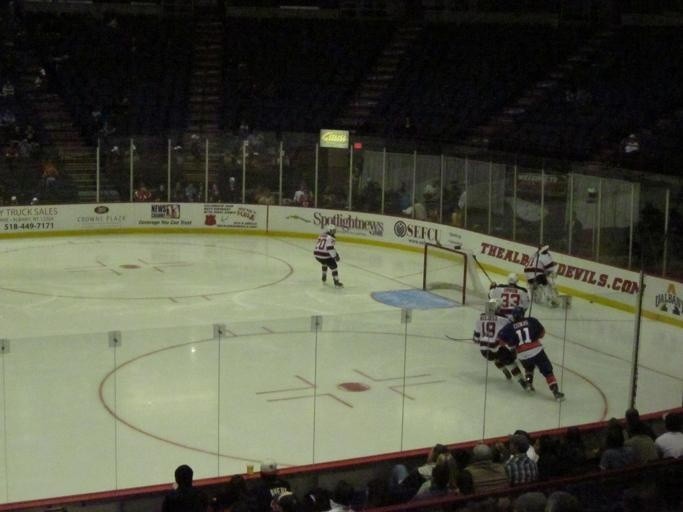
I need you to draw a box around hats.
[260,459,275,473]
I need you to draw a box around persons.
[2,67,61,192]
[523,243,561,308]
[488,273,530,318]
[162,410,683,511]
[471,298,530,391]
[133,175,468,230]
[495,305,564,399]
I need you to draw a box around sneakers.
[503,369,563,398]
[321,275,325,281]
[333,280,341,286]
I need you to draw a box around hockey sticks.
[444,333,473,342]
[454,245,493,285]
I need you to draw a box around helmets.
[327,224,335,234]
[512,306,524,321]
[485,299,500,315]
[508,273,519,284]
